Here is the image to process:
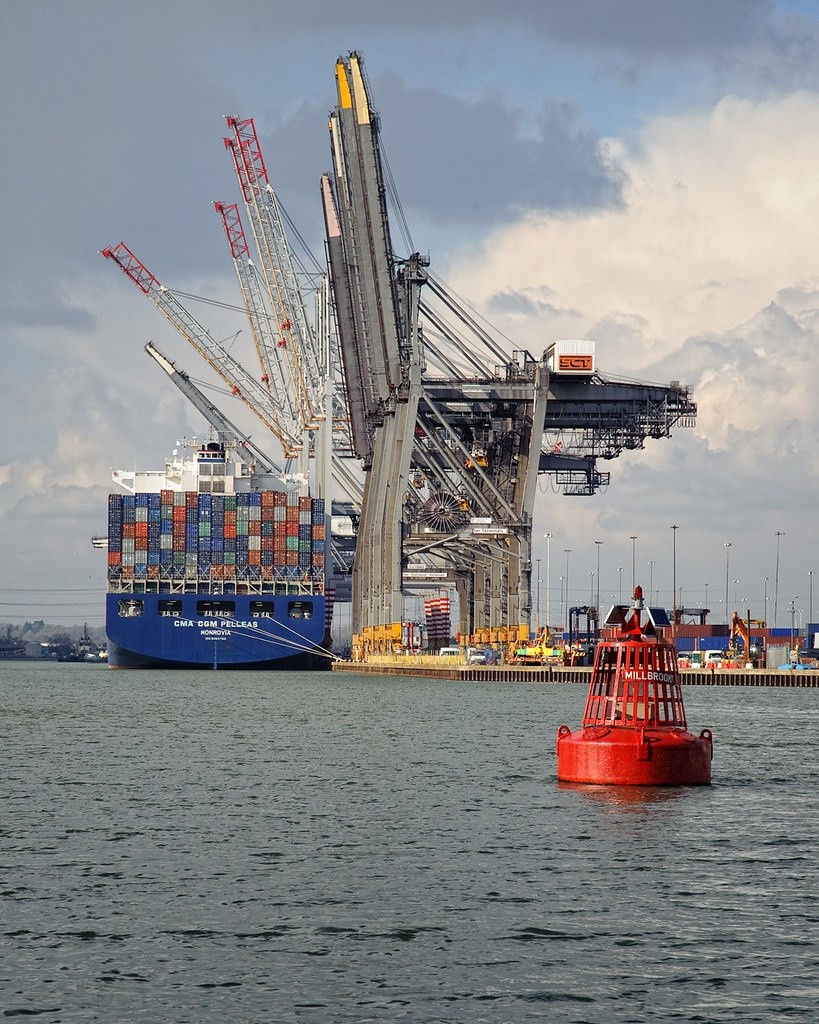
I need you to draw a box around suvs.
[678,652,692,659]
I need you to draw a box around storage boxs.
[107,490,324,594]
[617,702,656,720]
[530,623,819,671]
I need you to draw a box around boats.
[57,622,108,663]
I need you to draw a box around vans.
[705,650,722,664]
[440,647,459,663]
[468,648,486,665]
[541,656,564,666]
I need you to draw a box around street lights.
[594,540,604,625]
[560,576,564,627]
[647,561,655,607]
[682,540,772,627]
[670,524,679,636]
[589,570,594,605]
[774,531,785,627]
[630,535,638,593]
[808,570,814,623]
[544,532,557,626]
[618,567,624,605]
[536,559,542,628]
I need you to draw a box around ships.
[106,426,327,672]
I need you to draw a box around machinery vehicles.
[513,626,584,664]
